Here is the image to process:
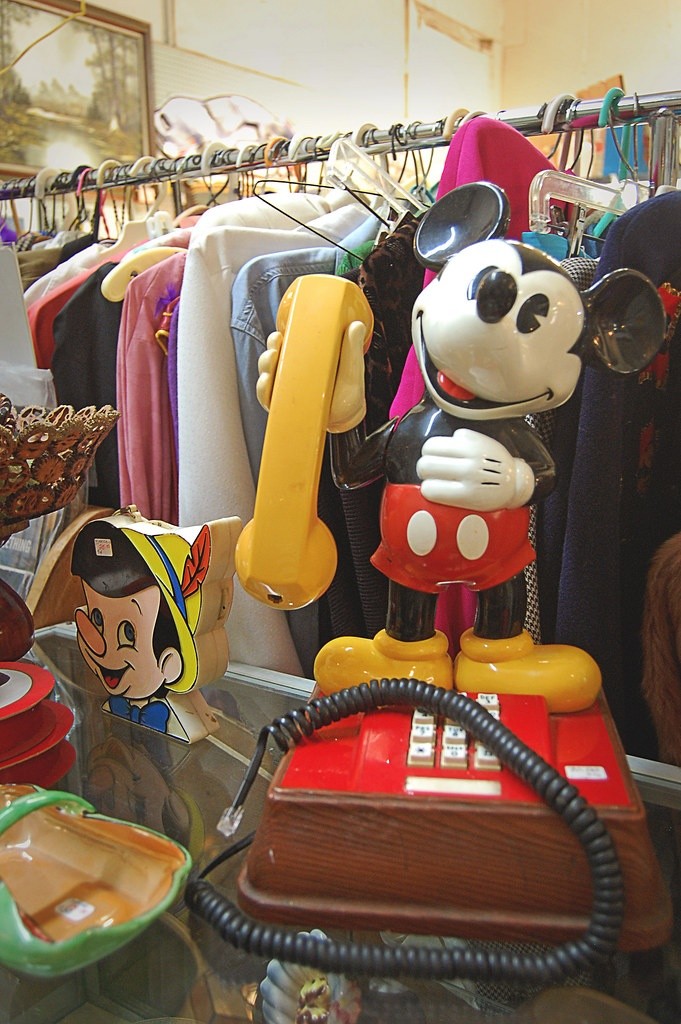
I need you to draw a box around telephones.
[234,274,375,609]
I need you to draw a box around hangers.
[0,87,677,272]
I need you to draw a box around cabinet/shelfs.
[0,622,680,1023]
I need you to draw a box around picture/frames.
[0,1,157,203]
